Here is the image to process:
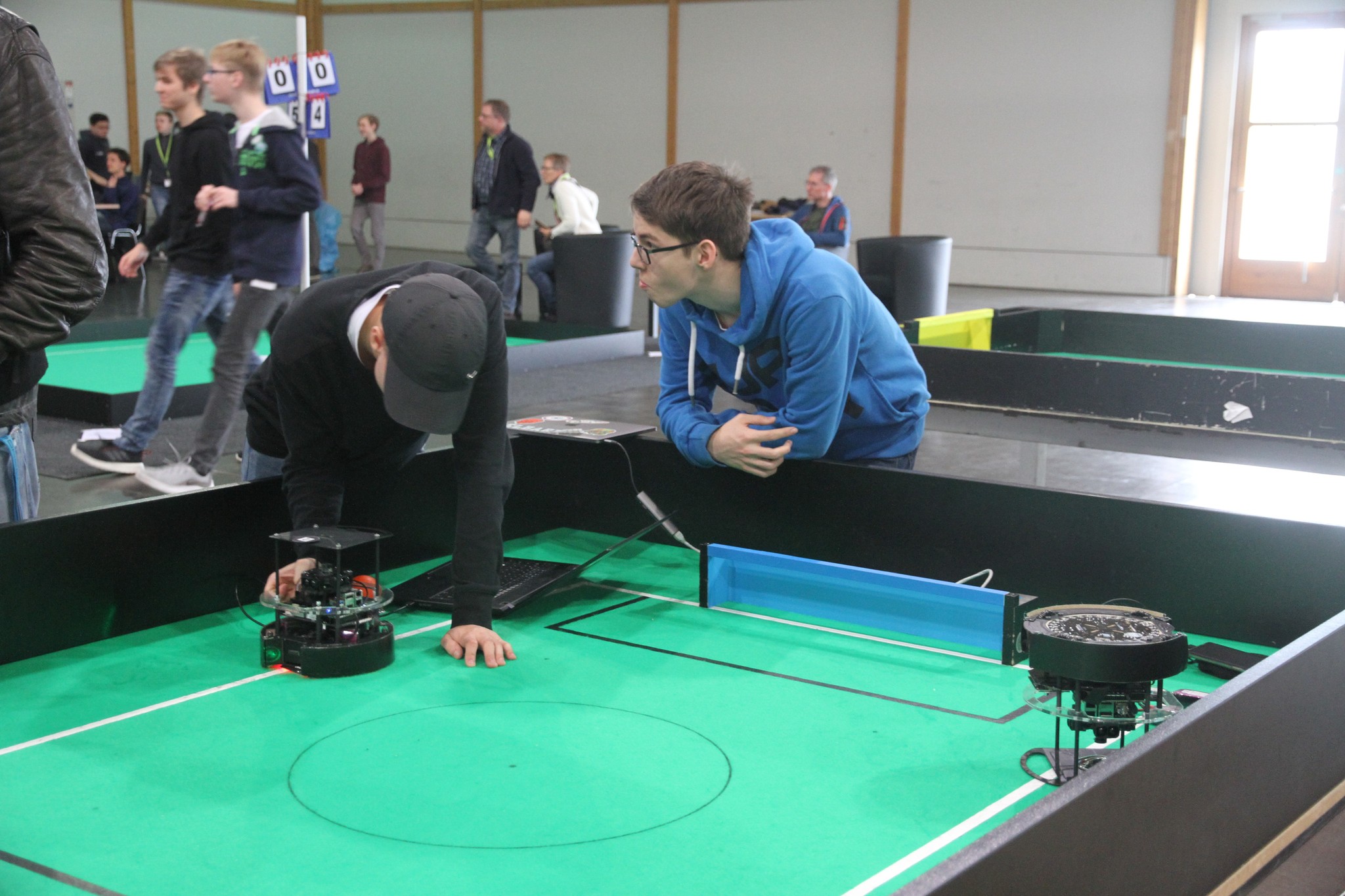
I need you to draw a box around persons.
[77,111,176,262]
[240,257,513,667]
[350,113,391,272]
[69,49,267,473]
[784,166,852,248]
[526,150,600,325]
[469,100,539,324]
[0,5,109,528]
[137,43,325,495]
[630,163,931,479]
[309,138,323,274]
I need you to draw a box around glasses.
[629,232,718,266]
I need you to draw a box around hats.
[383,272,487,435]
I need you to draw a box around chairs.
[100,194,147,279]
[534,224,636,330]
[856,236,953,325]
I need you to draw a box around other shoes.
[69,439,146,474]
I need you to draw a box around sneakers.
[136,439,215,494]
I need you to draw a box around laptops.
[384,516,668,614]
[505,414,658,444]
